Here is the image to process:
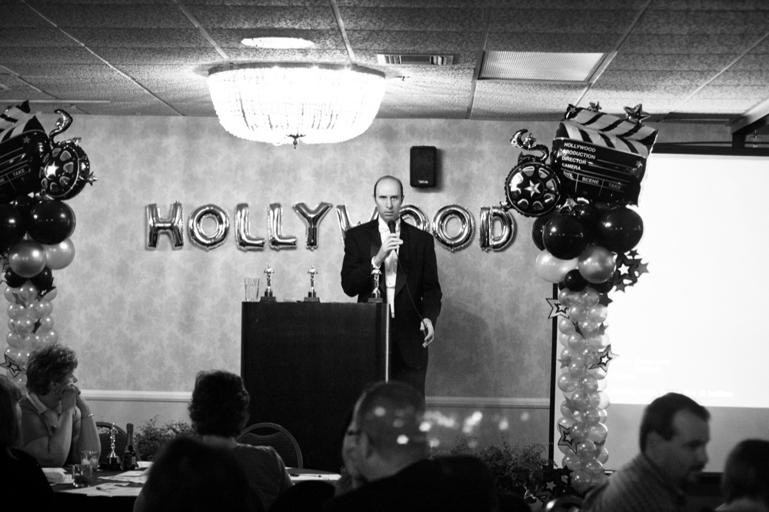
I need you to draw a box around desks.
[39,447,344,506]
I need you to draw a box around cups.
[244,278,259,302]
[80,448,99,482]
[72,464,90,488]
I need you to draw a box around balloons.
[1,252,58,395]
[38,239,76,271]
[12,238,48,277]
[553,271,609,494]
[588,206,644,255]
[26,198,75,248]
[1,202,28,250]
[543,211,588,262]
[531,215,551,251]
[535,247,580,284]
[578,244,617,284]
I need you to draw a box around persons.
[584,390,713,511]
[317,380,510,511]
[131,368,294,511]
[339,175,445,405]
[328,392,374,495]
[16,344,102,479]
[0,375,61,511]
[712,435,769,512]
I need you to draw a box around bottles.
[122,423,137,471]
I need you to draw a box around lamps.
[205,60,386,146]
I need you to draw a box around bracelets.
[81,411,94,421]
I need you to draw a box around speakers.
[410,146,437,188]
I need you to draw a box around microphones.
[388,220,397,251]
